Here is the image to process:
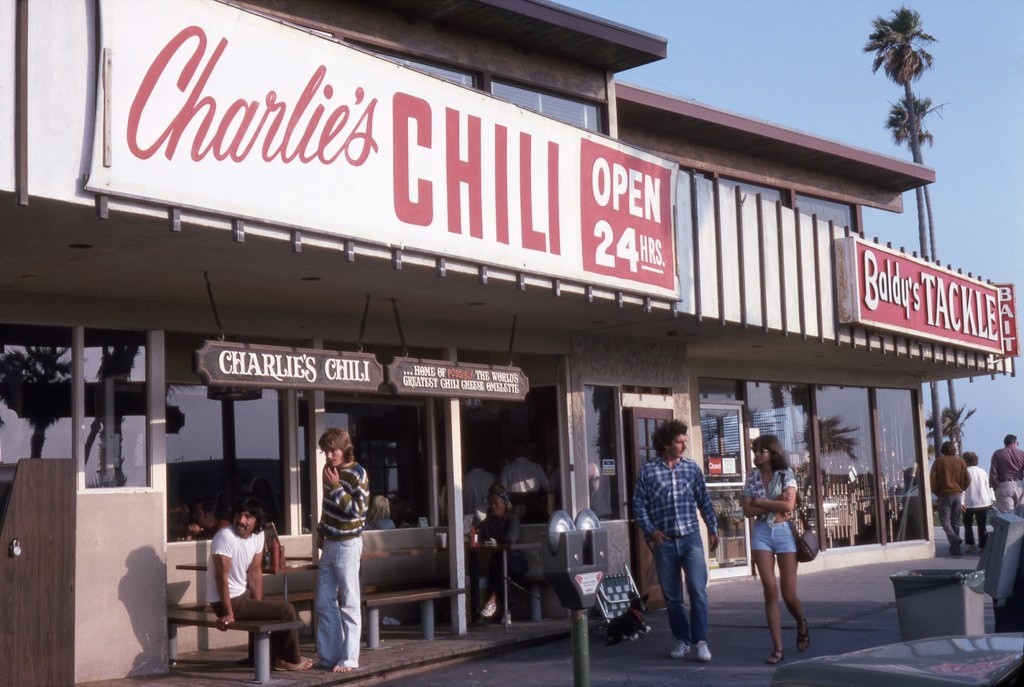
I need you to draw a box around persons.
[587,458,612,521]
[466,465,498,524]
[471,484,527,622]
[181,500,229,539]
[498,444,551,523]
[633,420,718,659]
[208,497,313,671]
[312,427,369,672]
[989,435,1024,514]
[930,441,971,554]
[362,494,395,530]
[961,451,996,553]
[744,435,810,665]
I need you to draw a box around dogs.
[605,591,651,646]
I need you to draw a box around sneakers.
[696,640,711,661]
[670,640,691,659]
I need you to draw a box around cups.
[469,534,478,543]
[436,533,447,548]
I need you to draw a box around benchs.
[164,568,551,683]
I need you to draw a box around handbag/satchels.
[796,525,819,562]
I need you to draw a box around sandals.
[768,650,784,664]
[797,618,809,652]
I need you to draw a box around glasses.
[1015,441,1019,446]
[753,447,768,453]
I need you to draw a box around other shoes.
[480,602,496,616]
[950,539,963,555]
[965,544,976,553]
[500,614,511,624]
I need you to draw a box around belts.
[999,478,1023,482]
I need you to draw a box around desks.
[176,539,545,650]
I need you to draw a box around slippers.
[275,657,315,671]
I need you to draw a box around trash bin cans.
[889,568,986,643]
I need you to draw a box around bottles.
[265,550,271,570]
